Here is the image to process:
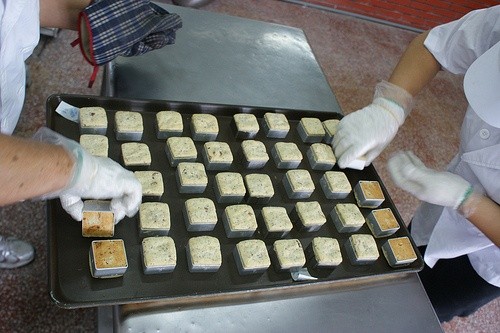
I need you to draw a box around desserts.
[77,106,417,279]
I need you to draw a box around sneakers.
[1,234,35,269]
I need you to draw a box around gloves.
[60,139,144,224]
[330,97,405,170]
[76,0,184,66]
[384,149,474,210]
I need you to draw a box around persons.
[331,4,500,323]
[0,0,145,269]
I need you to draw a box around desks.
[100,0,444,333]
